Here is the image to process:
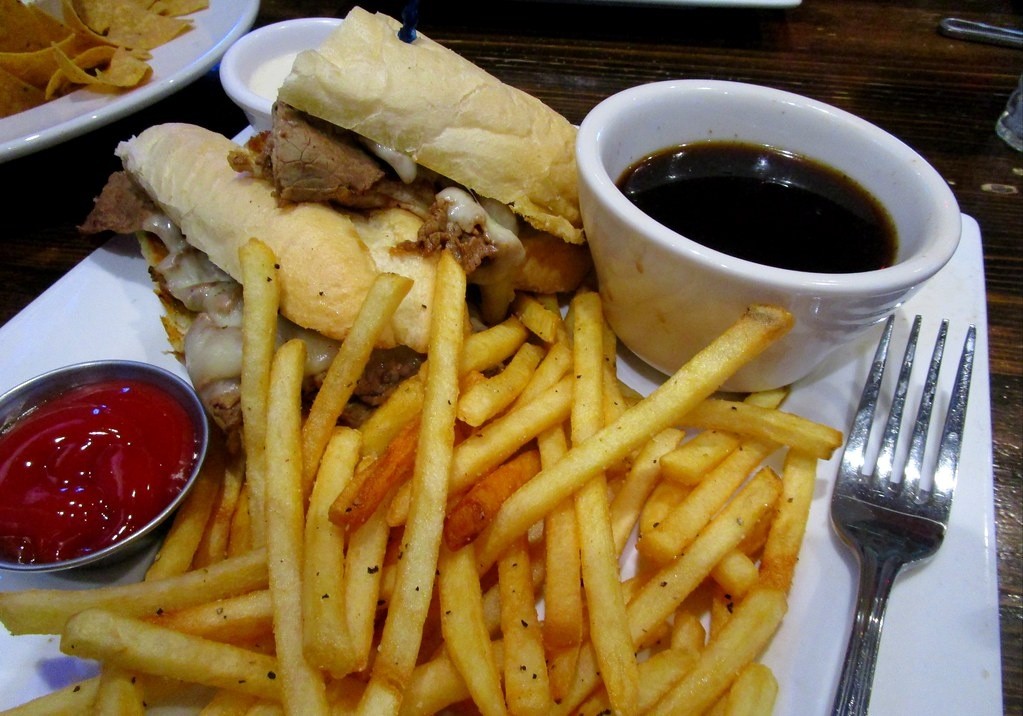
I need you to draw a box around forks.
[820,313,974,716]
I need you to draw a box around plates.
[0,1,261,162]
[1,208,1001,716]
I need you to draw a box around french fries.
[0,239,841,716]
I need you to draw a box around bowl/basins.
[580,78,965,393]
[219,16,345,135]
[1,359,209,576]
